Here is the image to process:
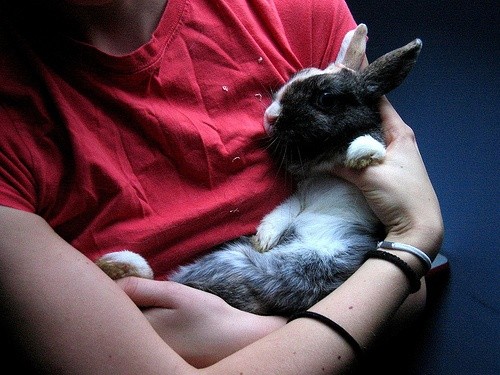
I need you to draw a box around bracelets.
[364,250,421,293]
[286,311,362,359]
[377,241,431,269]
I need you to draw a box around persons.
[0,0,445,375]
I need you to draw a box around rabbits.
[96,23,423,317]
